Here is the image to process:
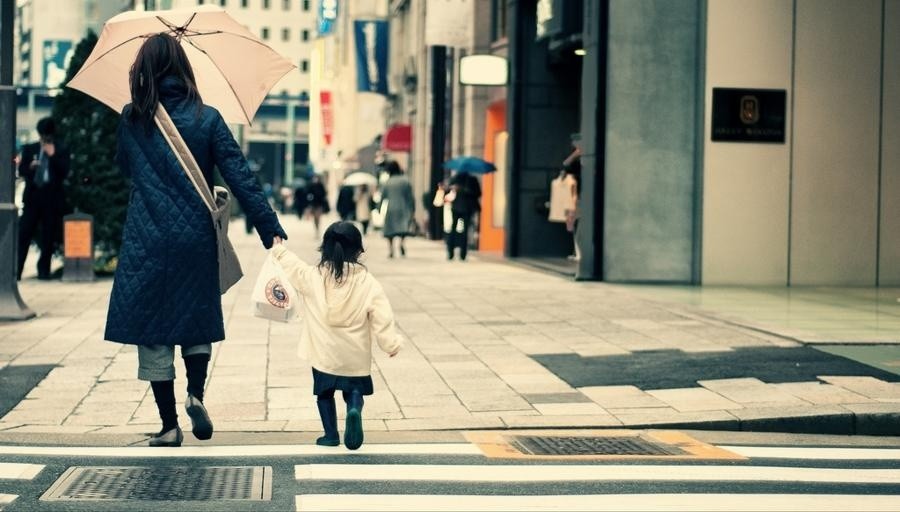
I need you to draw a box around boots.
[316,398,340,446]
[344,389,364,450]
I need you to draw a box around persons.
[296,171,330,240]
[377,160,416,258]
[337,186,355,220]
[354,185,371,235]
[270,220,401,449]
[17,116,68,280]
[103,31,284,443]
[438,171,482,260]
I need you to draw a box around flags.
[320,90,333,146]
[353,18,389,95]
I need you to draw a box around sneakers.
[150,425,183,446]
[185,394,213,440]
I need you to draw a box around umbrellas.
[342,171,379,186]
[441,155,497,174]
[64,3,298,127]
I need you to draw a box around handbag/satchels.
[211,185,244,295]
